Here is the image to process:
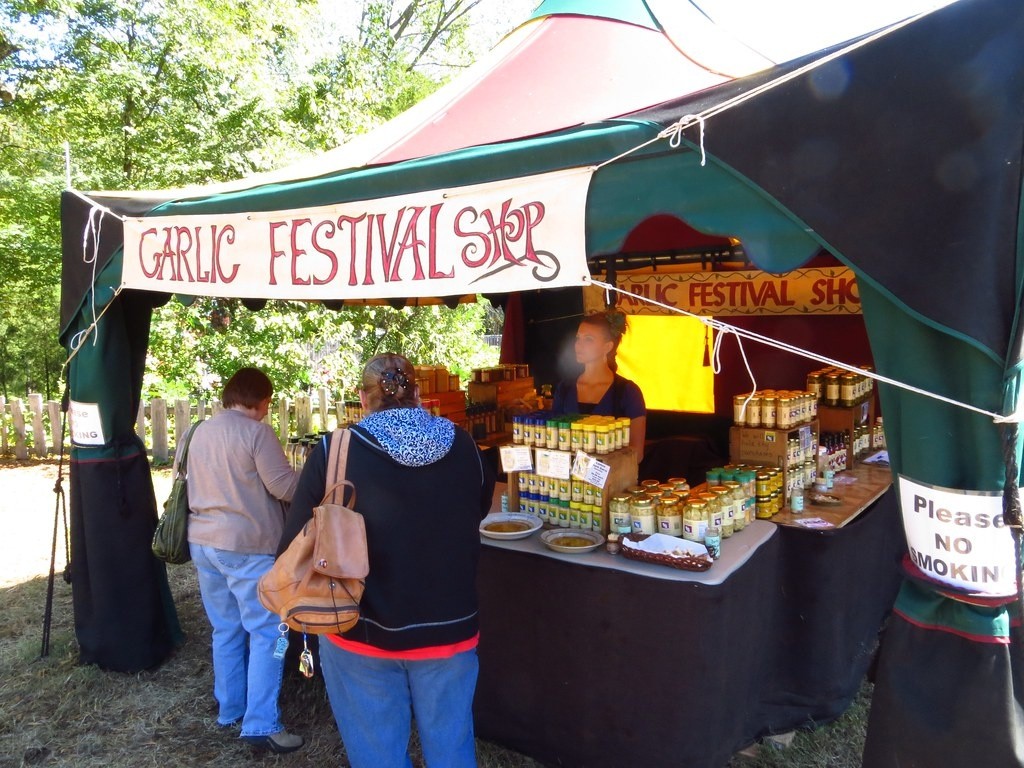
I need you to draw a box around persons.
[552,307,646,462]
[175,367,305,754]
[274,352,494,768]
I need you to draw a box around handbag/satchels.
[152,419,205,564]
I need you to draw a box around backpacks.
[257,430,370,635]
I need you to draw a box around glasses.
[266,397,274,409]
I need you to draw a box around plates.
[478,512,544,540]
[538,528,605,553]
[808,492,843,506]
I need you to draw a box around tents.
[61,0,1024,768]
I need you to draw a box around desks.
[471,518,791,768]
[770,446,907,731]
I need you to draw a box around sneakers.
[244,730,305,752]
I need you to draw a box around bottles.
[820,429,850,473]
[465,411,496,436]
